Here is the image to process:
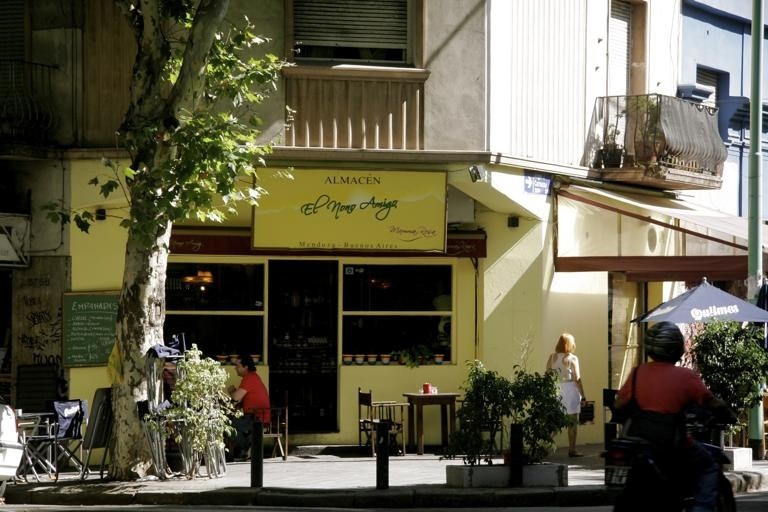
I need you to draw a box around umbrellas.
[629,276,768,369]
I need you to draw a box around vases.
[433,354,445,365]
[229,354,238,364]
[216,355,228,365]
[250,354,261,363]
[342,353,391,366]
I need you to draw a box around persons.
[228,353,272,462]
[614,321,737,512]
[545,332,586,457]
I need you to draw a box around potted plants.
[447,358,512,488]
[411,344,430,366]
[513,366,568,486]
[598,94,663,167]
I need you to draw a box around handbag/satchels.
[45,399,85,440]
[622,401,682,446]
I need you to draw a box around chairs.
[246,389,289,460]
[22,399,90,484]
[358,386,411,457]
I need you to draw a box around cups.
[14,408,22,417]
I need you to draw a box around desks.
[80,387,110,483]
[10,412,53,482]
[402,392,461,456]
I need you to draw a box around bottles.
[418,383,437,394]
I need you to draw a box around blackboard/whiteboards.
[61,290,121,368]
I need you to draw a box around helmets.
[644,322,684,357]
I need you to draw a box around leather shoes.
[233,455,251,461]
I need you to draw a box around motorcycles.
[602,392,745,512]
